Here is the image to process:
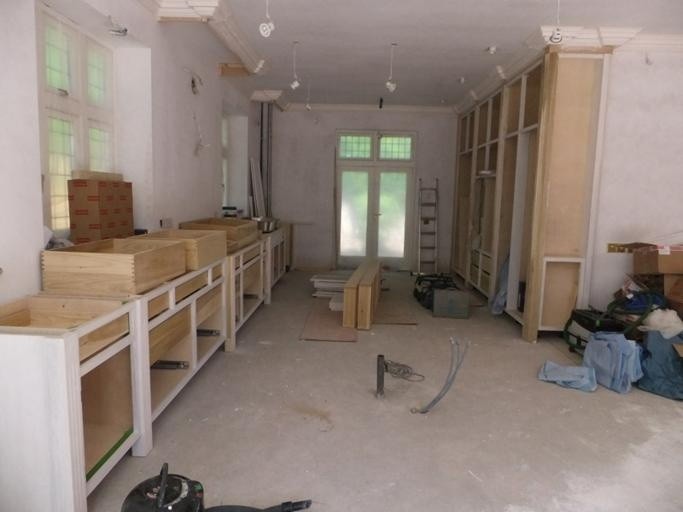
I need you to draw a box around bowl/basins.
[242,215,282,234]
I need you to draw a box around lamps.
[290,40,301,89]
[304,103,312,111]
[385,41,397,93]
[549,0,563,43]
[258,0,276,37]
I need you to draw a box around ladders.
[418,177,440,276]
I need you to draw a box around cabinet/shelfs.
[449,44,614,344]
[140,258,229,454]
[229,237,270,345]
[1,299,146,512]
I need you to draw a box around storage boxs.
[67,178,135,242]
[125,230,226,270]
[182,220,258,249]
[633,247,683,306]
[40,239,188,296]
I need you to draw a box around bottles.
[223,206,237,219]
